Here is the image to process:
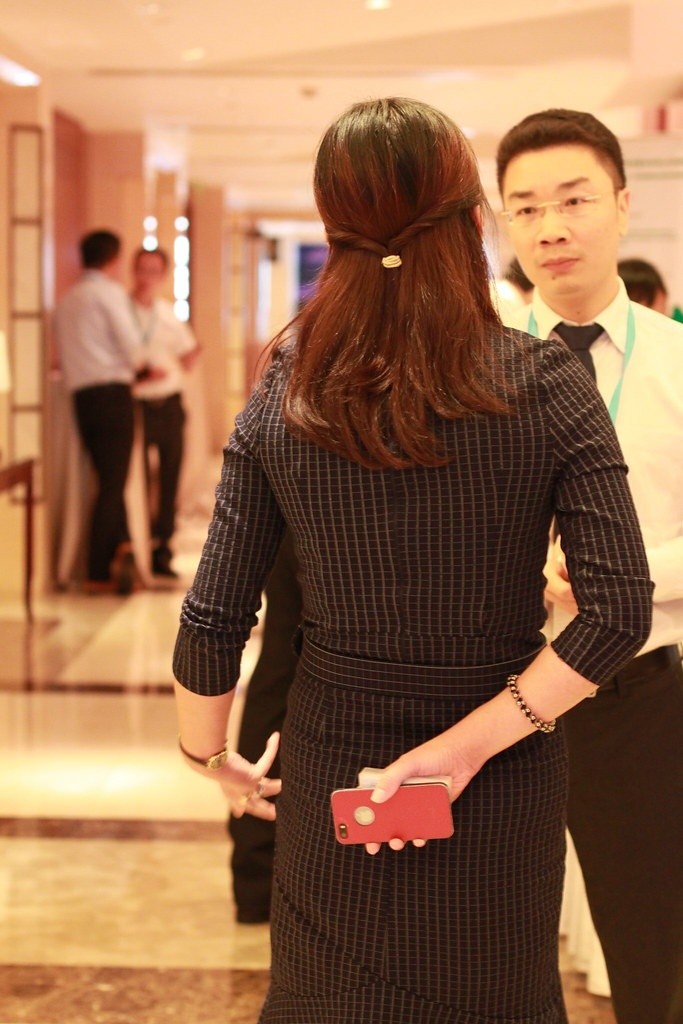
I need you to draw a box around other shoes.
[586,964,612,997]
[152,562,178,578]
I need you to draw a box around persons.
[488,109,682,1024]
[171,99,655,1024]
[55,229,202,599]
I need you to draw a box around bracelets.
[507,673,560,733]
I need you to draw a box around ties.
[554,320,604,384]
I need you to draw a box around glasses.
[501,190,617,225]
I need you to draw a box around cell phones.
[330,782,455,846]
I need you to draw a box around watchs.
[178,734,228,771]
